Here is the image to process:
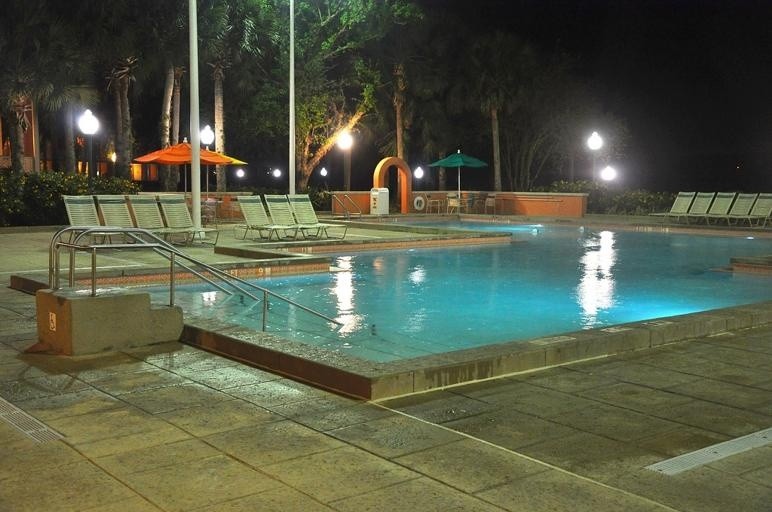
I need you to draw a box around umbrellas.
[426,150,488,212]
[205,145,249,201]
[133,137,233,202]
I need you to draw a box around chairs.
[647,191,772,228]
[424,192,496,214]
[61,194,349,252]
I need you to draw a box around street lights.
[334,129,354,192]
[198,123,216,204]
[76,106,99,195]
[587,128,604,184]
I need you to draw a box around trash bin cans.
[370,188,389,215]
